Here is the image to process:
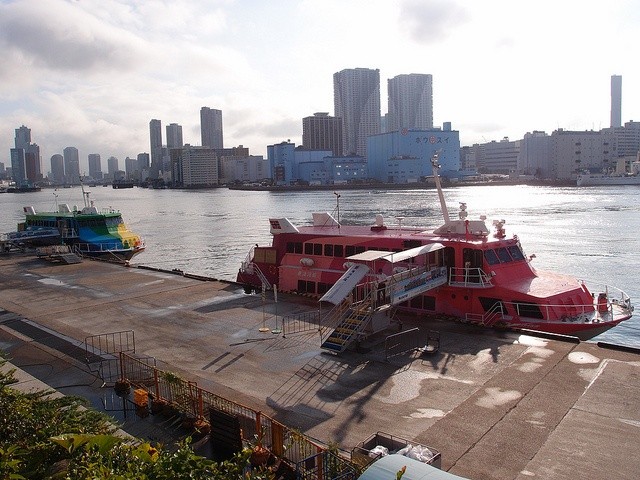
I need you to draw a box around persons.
[374,268,394,308]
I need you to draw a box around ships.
[238,149,634,342]
[18,190,146,260]
